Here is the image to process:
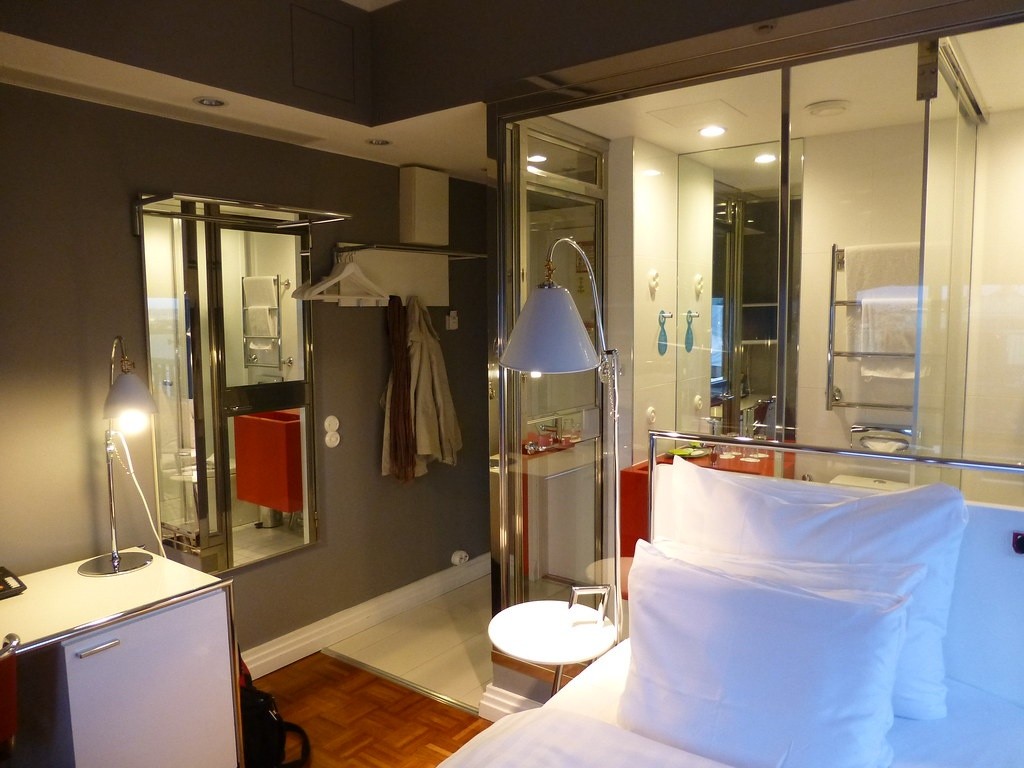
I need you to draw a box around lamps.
[77,334,169,578]
[495,229,625,639]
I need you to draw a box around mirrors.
[674,138,803,463]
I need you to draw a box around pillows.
[616,538,929,768]
[648,454,970,721]
[652,537,929,601]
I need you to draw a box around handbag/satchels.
[240,686,310,768]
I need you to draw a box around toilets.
[182,459,258,531]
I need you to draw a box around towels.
[161,448,198,469]
[844,240,920,426]
[861,298,917,379]
[206,453,216,469]
[243,276,284,385]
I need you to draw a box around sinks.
[234,407,303,513]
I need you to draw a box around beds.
[433,426,1024,768]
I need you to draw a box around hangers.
[291,244,391,301]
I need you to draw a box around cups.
[754,435,767,458]
[742,447,759,460]
[720,432,741,459]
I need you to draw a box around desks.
[487,584,618,697]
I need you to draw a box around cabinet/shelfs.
[0,544,246,768]
